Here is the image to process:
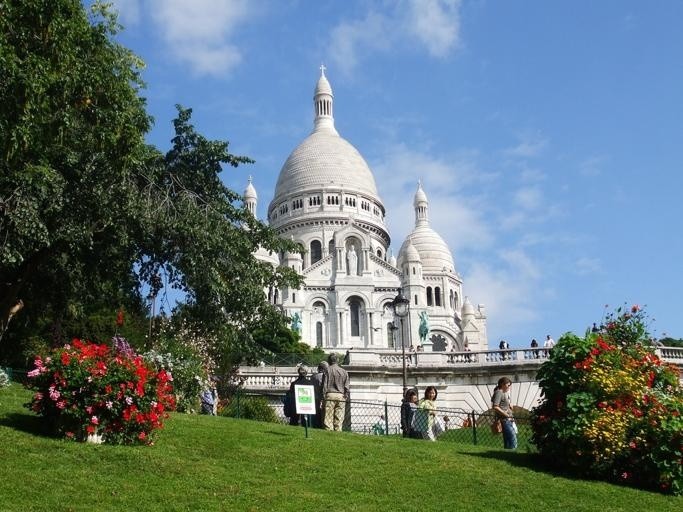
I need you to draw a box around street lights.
[392,288,410,397]
[147,289,154,339]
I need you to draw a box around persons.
[212,387,221,416]
[446,344,456,364]
[542,334,555,359]
[200,389,215,416]
[417,310,429,324]
[530,338,540,359]
[463,343,472,363]
[346,244,358,275]
[498,340,511,361]
[409,345,415,364]
[283,352,518,450]
[416,345,421,352]
